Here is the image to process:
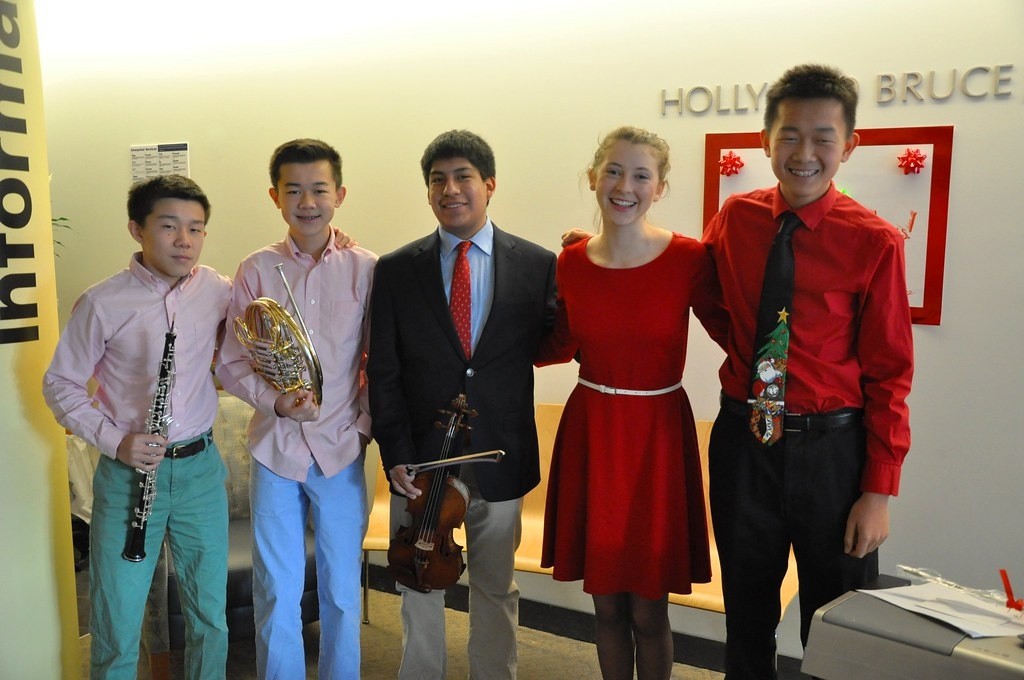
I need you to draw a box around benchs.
[363,402,799,676]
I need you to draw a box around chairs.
[141,397,322,680]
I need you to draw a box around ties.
[749,207,804,447]
[448,241,472,363]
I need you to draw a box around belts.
[577,377,683,396]
[720,390,863,433]
[164,429,213,458]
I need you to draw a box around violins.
[387,394,477,596]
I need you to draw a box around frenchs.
[230,262,326,408]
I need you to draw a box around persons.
[366,129,582,680]
[534,124,730,680]
[42,176,360,680]
[214,138,381,680]
[559,63,915,680]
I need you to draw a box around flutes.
[121,312,180,563]
